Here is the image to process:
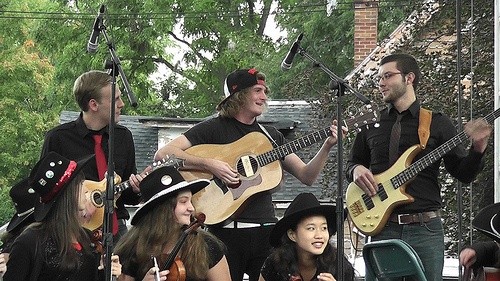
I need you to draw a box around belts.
[222,221,276,229]
[388,210,441,225]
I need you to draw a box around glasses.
[378,72,408,82]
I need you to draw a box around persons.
[114,166,232,281]
[345,54,491,281]
[0,152,122,281]
[40,71,152,246]
[155,69,348,281]
[258,192,356,281]
[458,239,500,270]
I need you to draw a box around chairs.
[362,239,427,281]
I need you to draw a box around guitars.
[78,154,181,231]
[178,102,382,231]
[346,107,500,236]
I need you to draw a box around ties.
[388,109,409,169]
[94,134,118,236]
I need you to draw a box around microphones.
[86,4,104,54]
[280,31,303,71]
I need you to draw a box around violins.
[91,230,117,281]
[151,212,206,281]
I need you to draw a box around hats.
[6,176,35,233]
[28,151,96,222]
[472,202,500,244]
[131,165,211,226]
[270,193,347,248]
[216,68,264,111]
